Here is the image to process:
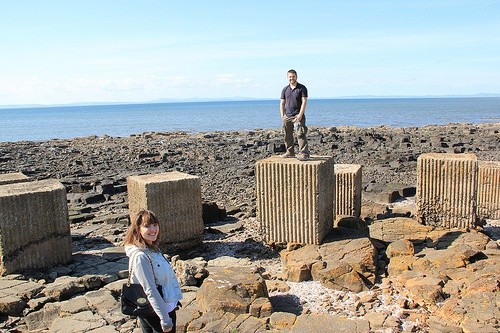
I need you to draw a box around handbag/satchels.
[120,250,164,317]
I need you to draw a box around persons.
[280,69,310,161]
[124,210,184,333]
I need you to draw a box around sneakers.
[280,152,295,158]
[297,152,309,161]
